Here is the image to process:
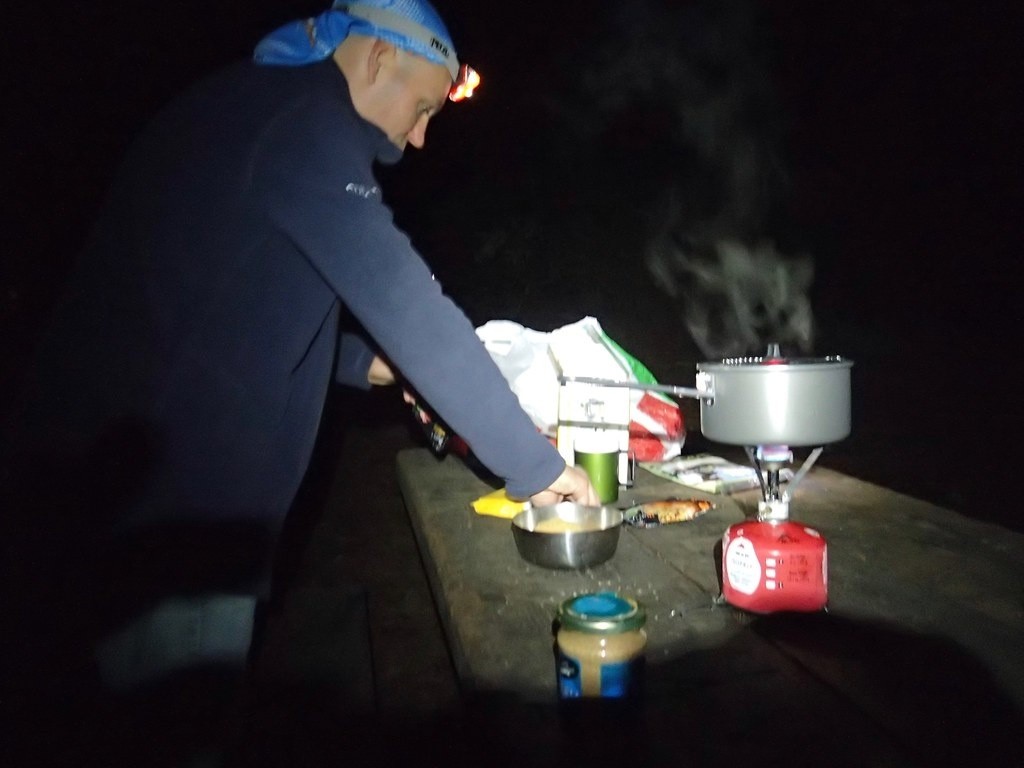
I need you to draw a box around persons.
[0,1,600,767]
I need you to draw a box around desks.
[398,442,1023,768]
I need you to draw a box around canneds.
[555,591,648,719]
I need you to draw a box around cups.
[574,437,635,503]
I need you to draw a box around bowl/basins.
[511,500,624,572]
